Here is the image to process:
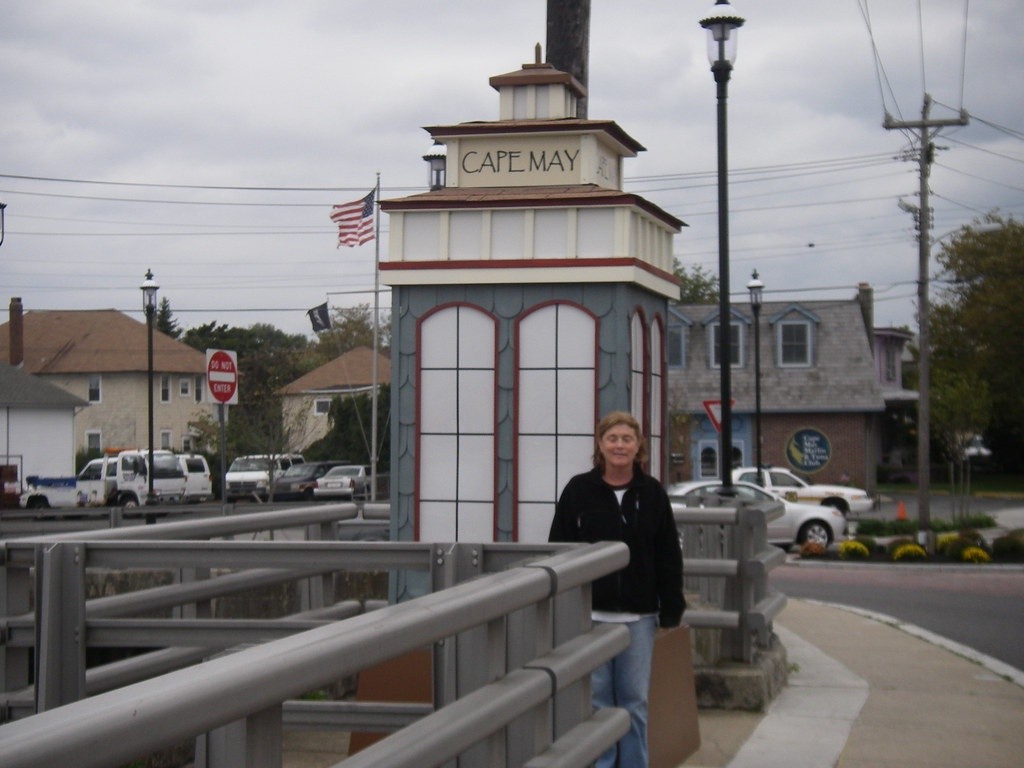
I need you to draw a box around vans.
[224,454,305,503]
[76,453,212,508]
[275,461,348,503]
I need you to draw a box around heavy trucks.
[77,449,186,518]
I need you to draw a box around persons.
[548,412,686,768]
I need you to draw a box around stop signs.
[206,348,238,405]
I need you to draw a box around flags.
[331,191,375,248]
[308,303,330,331]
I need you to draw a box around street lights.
[139,269,160,524]
[699,0,744,498]
[748,268,767,492]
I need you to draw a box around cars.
[667,481,850,553]
[312,465,390,502]
[730,465,872,522]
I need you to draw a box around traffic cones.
[896,501,908,521]
